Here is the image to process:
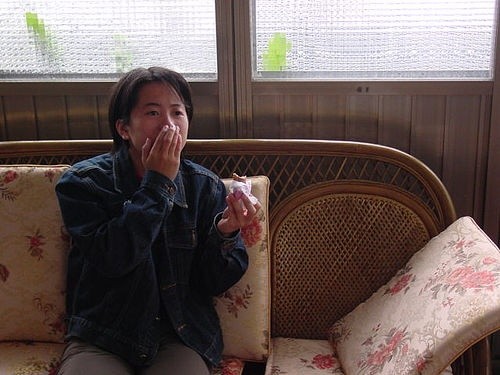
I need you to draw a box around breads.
[232,173,246,184]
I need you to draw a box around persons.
[54,65,263,375]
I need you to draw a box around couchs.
[0,137,500,375]
